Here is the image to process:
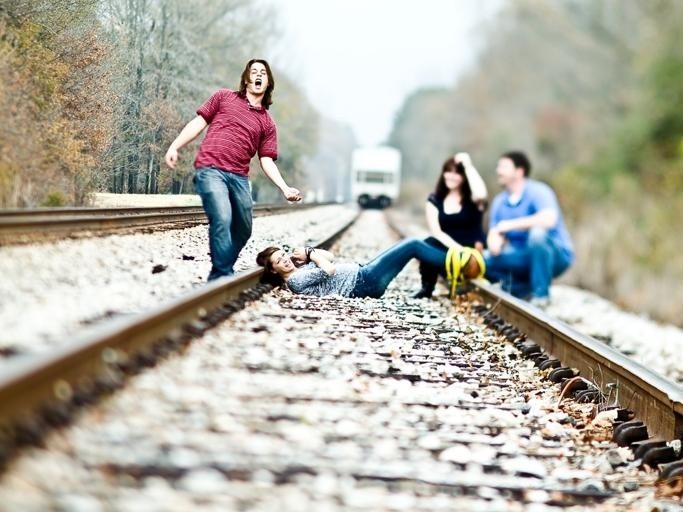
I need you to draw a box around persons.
[479,153,575,309]
[256,236,487,299]
[163,58,305,282]
[409,150,490,301]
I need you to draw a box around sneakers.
[410,288,434,299]
[529,296,551,310]
[463,241,483,278]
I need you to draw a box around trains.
[350,142,402,209]
